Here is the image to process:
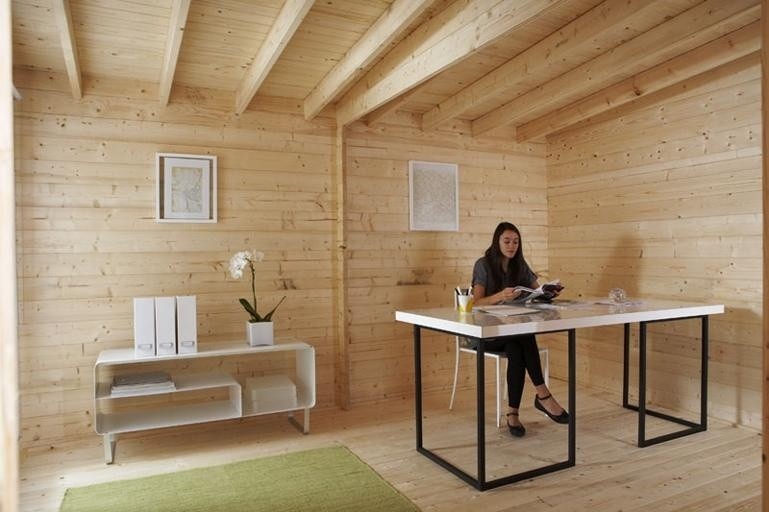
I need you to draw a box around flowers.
[229,248,288,321]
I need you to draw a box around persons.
[468,222,568,438]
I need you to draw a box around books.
[510,278,565,303]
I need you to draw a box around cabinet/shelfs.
[93,337,316,464]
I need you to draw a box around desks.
[395,299,726,491]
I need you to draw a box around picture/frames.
[155,153,218,224]
[408,160,460,232]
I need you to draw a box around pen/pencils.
[455,285,472,296]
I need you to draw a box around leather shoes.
[506,413,526,436]
[535,393,569,423]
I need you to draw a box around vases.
[245,319,273,347]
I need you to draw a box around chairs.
[448,290,549,428]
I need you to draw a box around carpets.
[59,444,422,511]
